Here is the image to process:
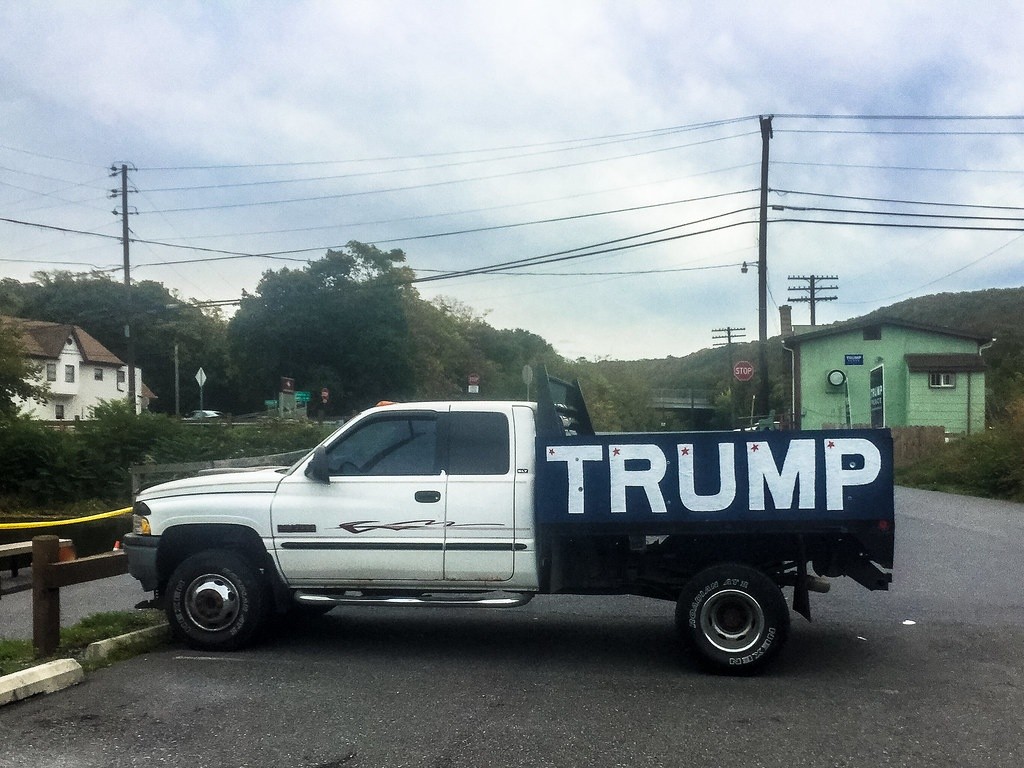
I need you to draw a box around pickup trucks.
[127,363,897,677]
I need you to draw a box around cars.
[179,410,227,422]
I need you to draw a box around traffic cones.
[112,540,120,553]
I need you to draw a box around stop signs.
[733,361,755,382]
